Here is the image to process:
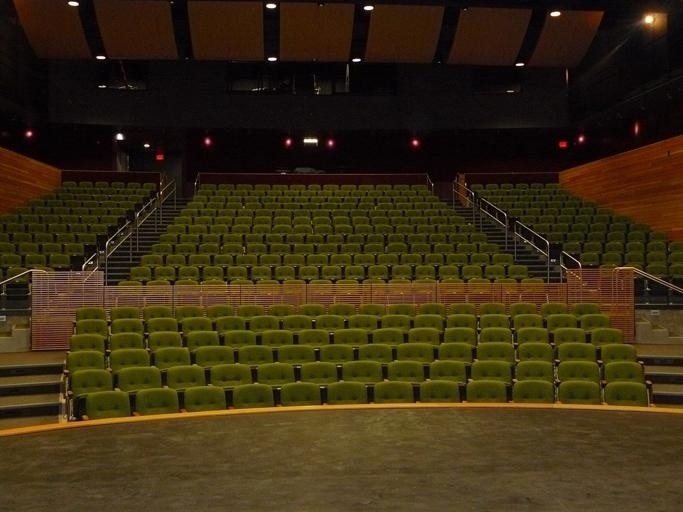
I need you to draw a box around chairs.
[0,179,682,303]
[57,302,655,426]
